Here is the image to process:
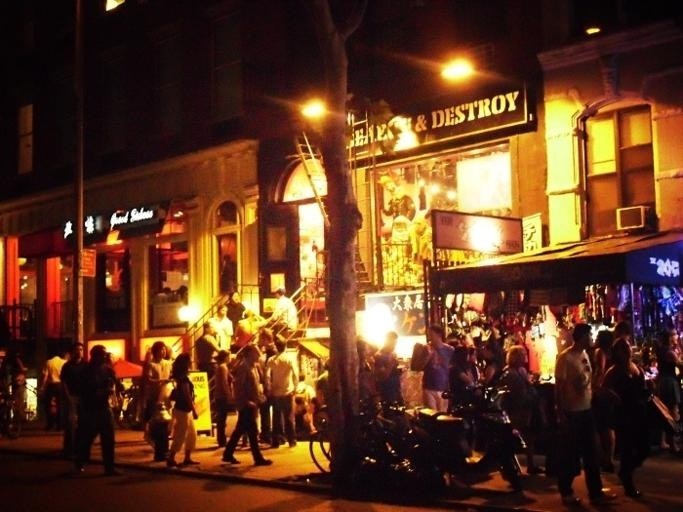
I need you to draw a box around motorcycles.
[384,358,528,497]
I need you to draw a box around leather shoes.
[255,458,273,466]
[183,459,200,466]
[167,460,180,467]
[221,455,242,465]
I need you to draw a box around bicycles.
[307,391,403,482]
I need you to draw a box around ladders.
[294,129,377,292]
[351,109,374,283]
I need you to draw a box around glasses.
[583,358,591,372]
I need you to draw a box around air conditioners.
[616,206,655,234]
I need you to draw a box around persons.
[38,254,299,476]
[357,320,683,505]
[380,187,416,271]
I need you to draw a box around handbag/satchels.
[511,369,537,410]
[645,394,677,435]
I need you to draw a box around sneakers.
[104,468,123,477]
[589,493,617,506]
[561,495,580,505]
